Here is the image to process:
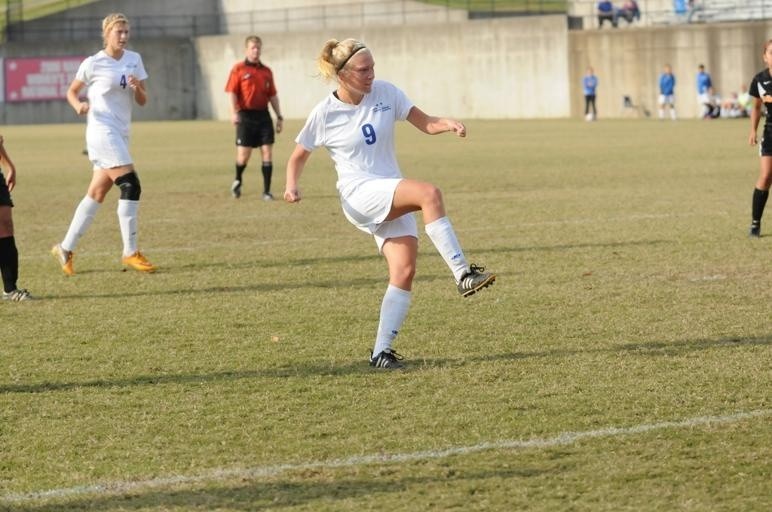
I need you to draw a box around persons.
[581,67,598,122]
[222,36,284,200]
[614,0,640,25]
[697,63,766,119]
[748,39,772,236]
[656,64,677,120]
[594,0,614,27]
[50,14,155,275]
[283,38,496,369]
[1,124,33,301]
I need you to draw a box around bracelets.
[277,115,283,120]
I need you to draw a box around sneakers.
[749,224,760,240]
[120,249,157,273]
[230,180,243,202]
[50,243,76,276]
[262,192,274,202]
[368,347,407,371]
[2,288,33,302]
[455,263,496,298]
